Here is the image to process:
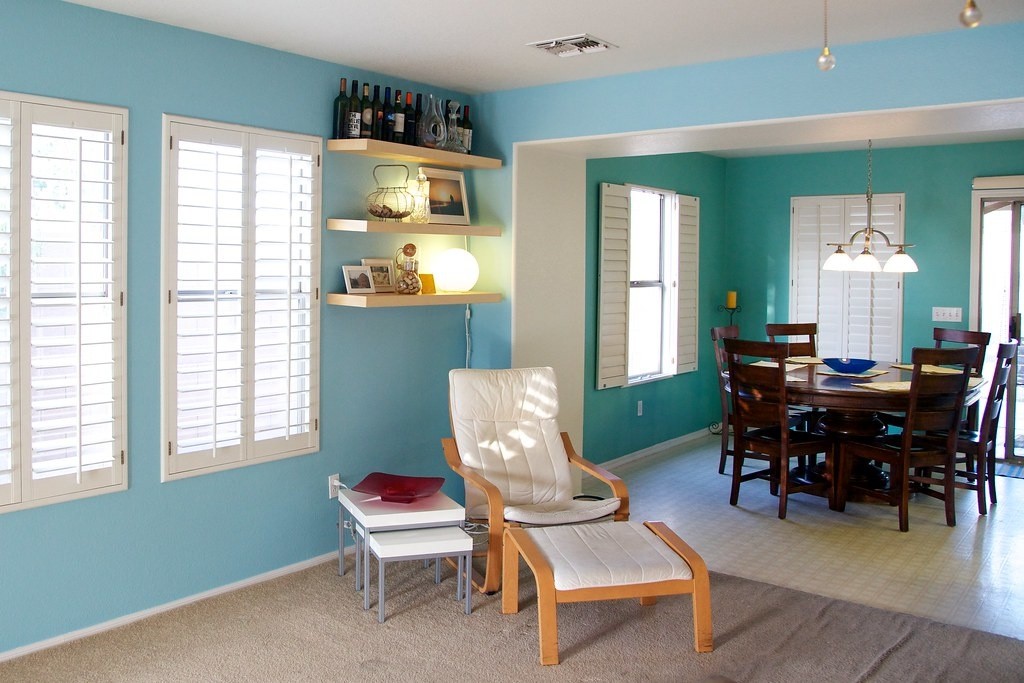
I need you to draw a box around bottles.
[393,89,404,144]
[403,91,415,146]
[382,86,395,142]
[361,82,372,139]
[348,80,360,139]
[409,174,431,224]
[415,92,473,154]
[371,85,383,140]
[333,78,348,140]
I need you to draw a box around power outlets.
[638,400,642,416]
[328,474,341,500]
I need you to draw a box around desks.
[337,488,466,612]
[721,359,988,513]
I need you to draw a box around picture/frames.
[342,266,376,294]
[361,259,396,293]
[419,167,471,226]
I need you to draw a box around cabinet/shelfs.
[325,138,502,308]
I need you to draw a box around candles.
[727,291,737,308]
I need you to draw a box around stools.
[501,521,714,665]
[354,523,474,624]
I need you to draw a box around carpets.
[973,461,1024,479]
[0,525,1024,683]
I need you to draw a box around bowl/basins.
[822,358,877,375]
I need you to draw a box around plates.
[351,472,446,504]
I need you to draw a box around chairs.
[710,325,808,483]
[766,322,828,466]
[879,328,993,483]
[912,338,1019,516]
[722,335,840,521]
[441,367,630,595]
[839,344,979,532]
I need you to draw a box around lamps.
[822,139,919,272]
